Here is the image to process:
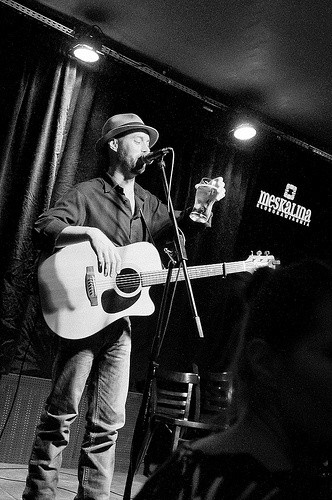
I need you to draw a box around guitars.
[37,241,281,339]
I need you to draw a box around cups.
[189,177,219,223]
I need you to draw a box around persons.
[23,113,226,500]
[131,264,332,500]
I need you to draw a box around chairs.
[135,370,225,479]
[192,365,240,428]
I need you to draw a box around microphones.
[141,147,169,164]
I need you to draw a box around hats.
[95,113,158,152]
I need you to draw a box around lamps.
[65,27,104,69]
